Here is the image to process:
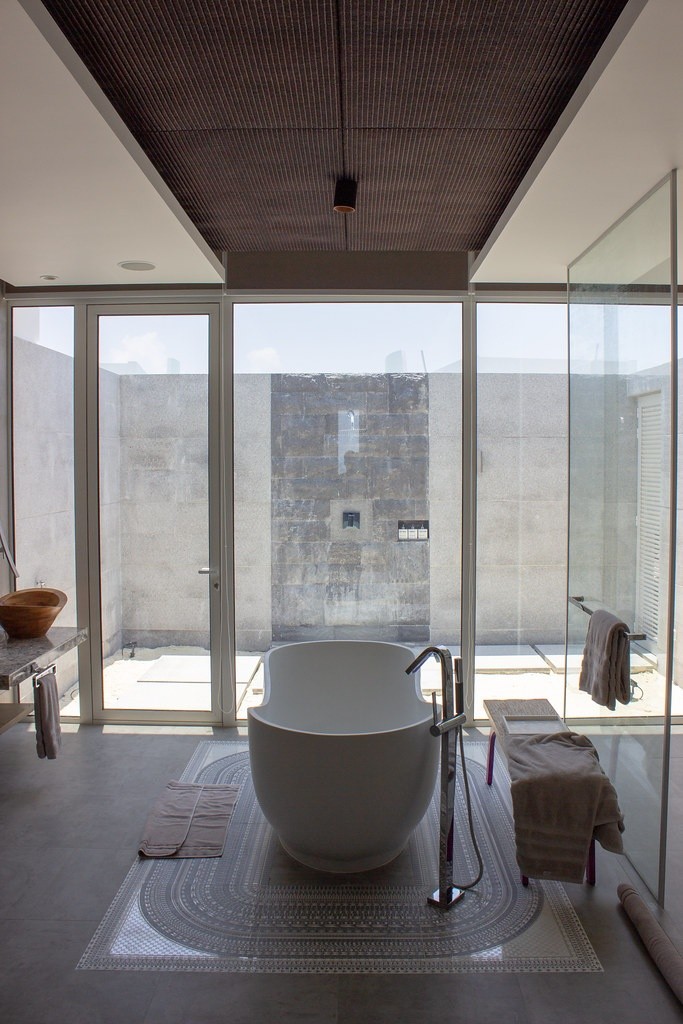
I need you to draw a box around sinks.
[0,584,68,642]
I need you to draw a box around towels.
[138,777,242,860]
[577,606,633,713]
[615,881,683,1006]
[507,731,629,887]
[33,673,64,762]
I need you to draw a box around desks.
[1,623,89,737]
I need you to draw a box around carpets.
[138,780,242,859]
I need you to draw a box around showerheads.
[452,654,466,716]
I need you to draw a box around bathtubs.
[245,637,442,876]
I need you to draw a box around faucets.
[0,527,22,579]
[406,643,455,682]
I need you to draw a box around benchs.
[483,696,613,888]
